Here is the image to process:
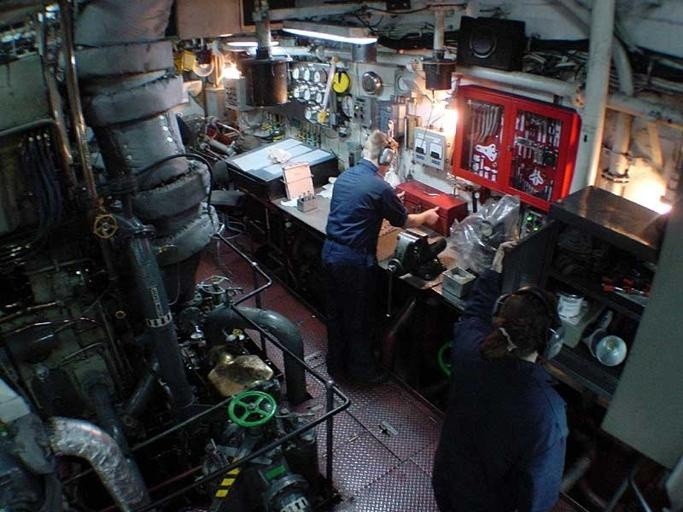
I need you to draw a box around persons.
[317,128,441,387]
[430,240,569,512]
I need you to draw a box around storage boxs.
[395,180,467,237]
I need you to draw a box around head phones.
[492,286,566,361]
[378,137,394,166]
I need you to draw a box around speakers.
[456,15,527,71]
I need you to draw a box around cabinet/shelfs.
[495,187,666,398]
[226,138,340,277]
[452,86,580,213]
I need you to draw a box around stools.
[206,191,245,239]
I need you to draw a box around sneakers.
[360,369,397,386]
